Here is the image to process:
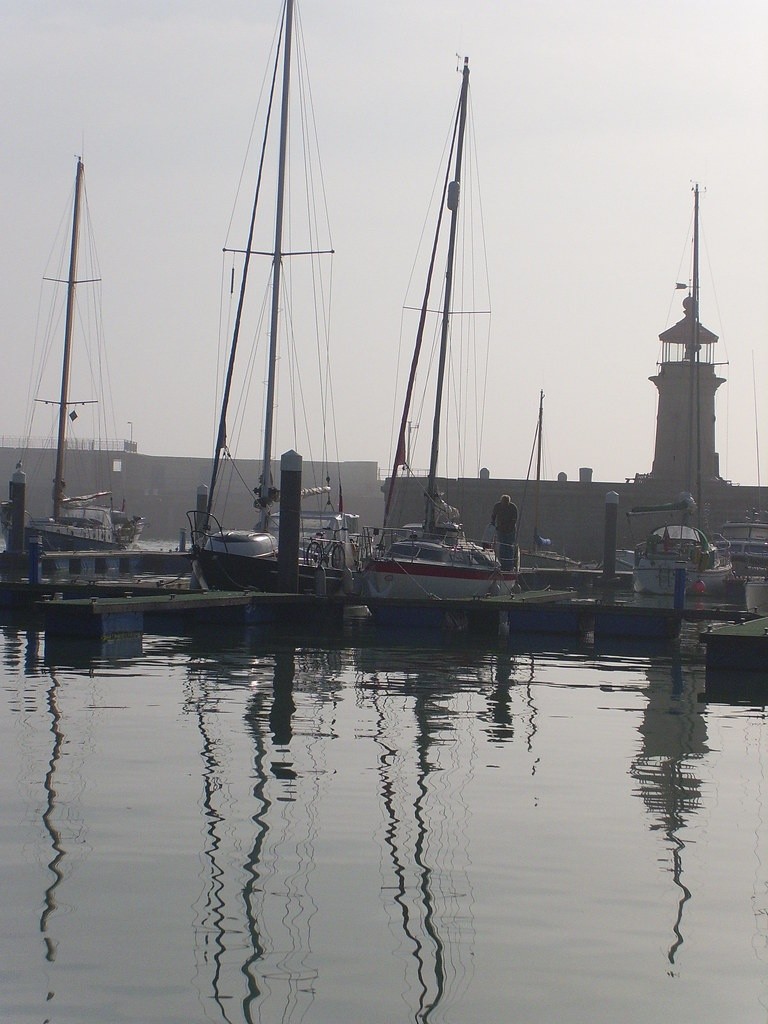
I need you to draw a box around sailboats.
[0,2,768,630]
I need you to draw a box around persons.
[490,494,519,572]
